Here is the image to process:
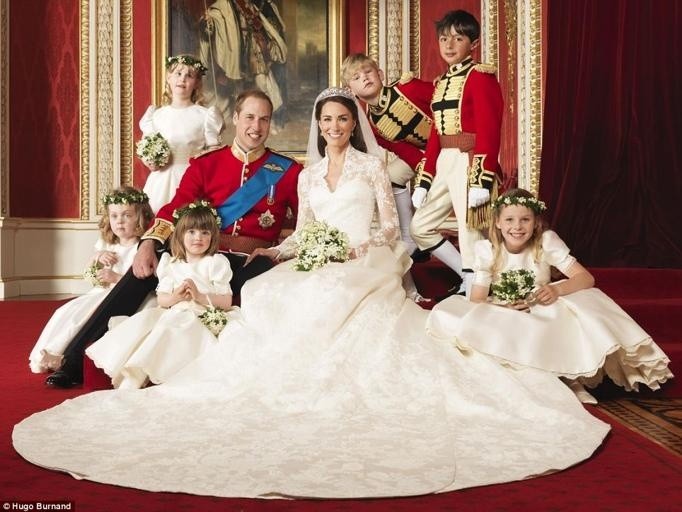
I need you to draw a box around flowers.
[490,195,549,212]
[135,132,172,170]
[85,265,106,287]
[289,221,352,273]
[199,307,229,339]
[166,54,208,78]
[490,268,538,306]
[100,192,150,205]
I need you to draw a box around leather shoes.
[45,372,82,388]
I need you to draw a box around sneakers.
[569,380,598,406]
[434,285,466,303]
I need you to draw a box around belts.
[219,233,279,255]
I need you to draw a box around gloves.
[411,186,428,211]
[468,187,490,210]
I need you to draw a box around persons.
[82,201,240,391]
[134,8,505,295]
[429,186,675,407]
[28,187,154,375]
[11,86,612,500]
[193,1,289,137]
[43,88,305,390]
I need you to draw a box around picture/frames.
[148,0,349,167]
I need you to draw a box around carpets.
[581,388,681,474]
